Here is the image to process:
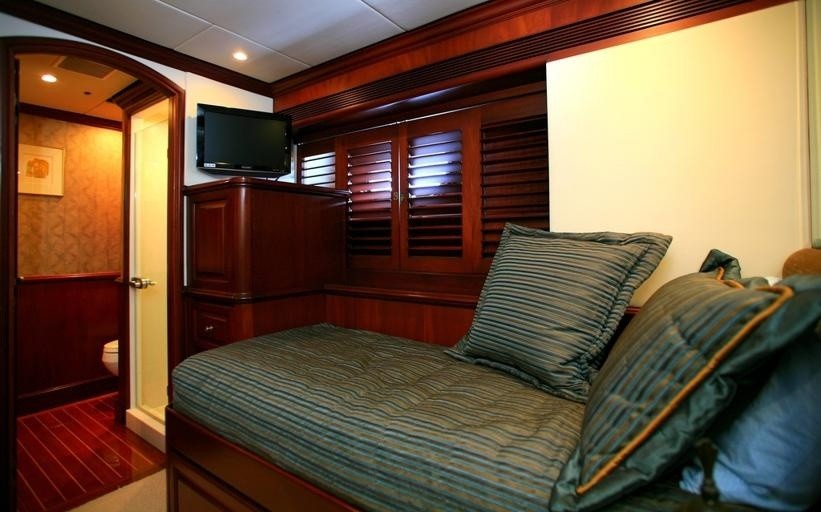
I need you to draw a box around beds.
[166,322,821,512]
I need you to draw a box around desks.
[184,273,483,357]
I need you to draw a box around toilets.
[100,339,119,377]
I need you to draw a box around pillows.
[681,344,821,512]
[547,248,821,512]
[444,221,674,406]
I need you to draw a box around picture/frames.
[17,142,65,198]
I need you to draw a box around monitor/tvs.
[196,103,291,178]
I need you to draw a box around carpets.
[48,460,167,512]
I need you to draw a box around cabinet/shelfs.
[184,177,351,295]
